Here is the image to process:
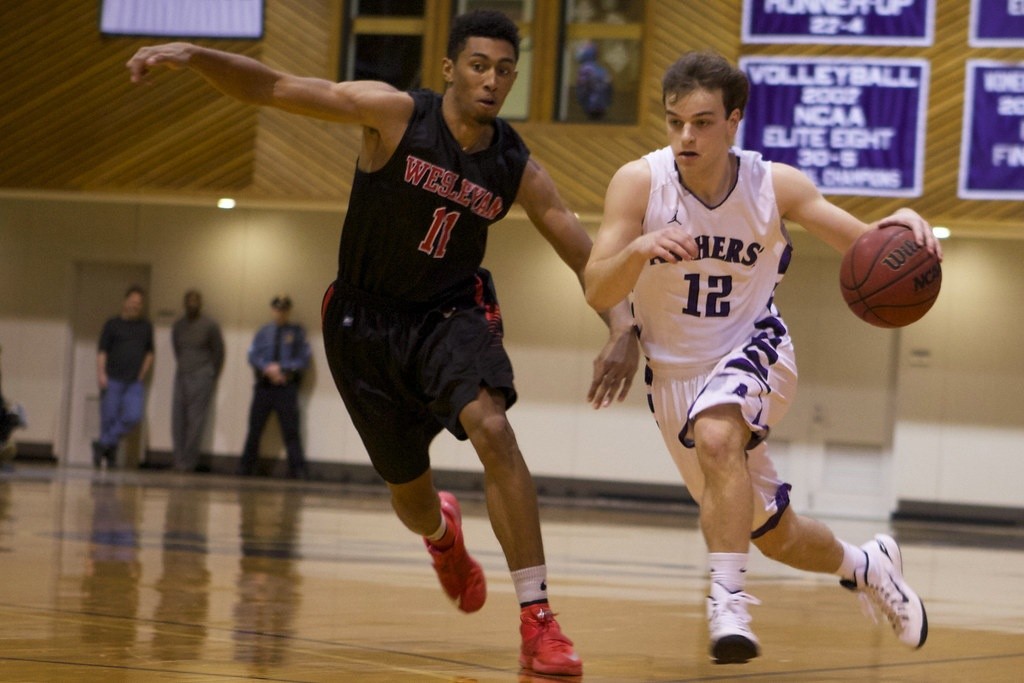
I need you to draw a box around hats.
[270,296,293,310]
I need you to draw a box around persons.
[234,293,312,479]
[128,9,639,678]
[93,286,156,470]
[585,51,944,667]
[161,291,226,477]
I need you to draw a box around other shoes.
[90,439,117,470]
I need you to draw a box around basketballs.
[838,223,943,331]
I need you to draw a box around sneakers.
[518,604,583,676]
[706,580,761,665]
[423,492,487,613]
[839,534,928,650]
